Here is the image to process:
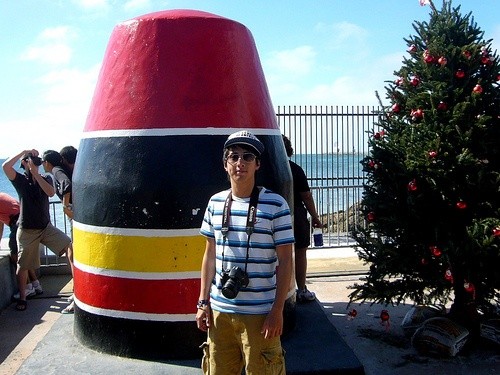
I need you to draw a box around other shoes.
[15,301,28,312]
[67,293,75,304]
[297,286,316,304]
[61,301,74,314]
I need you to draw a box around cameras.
[22,153,42,166]
[220,265,249,299]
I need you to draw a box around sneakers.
[13,285,43,299]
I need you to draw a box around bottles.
[312,224,323,248]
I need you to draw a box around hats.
[224,130,265,155]
[20,150,37,169]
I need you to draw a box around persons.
[0,192,44,300]
[195,130,297,375]
[282,134,324,302]
[59,145,77,171]
[42,150,72,241]
[2,149,74,311]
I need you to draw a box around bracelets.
[62,204,71,209]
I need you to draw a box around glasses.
[227,153,256,162]
